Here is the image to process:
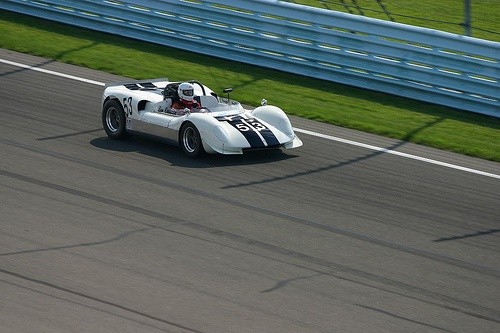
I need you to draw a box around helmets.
[178,83,194,102]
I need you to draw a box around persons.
[167,82,201,115]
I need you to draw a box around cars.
[100,77,304,158]
